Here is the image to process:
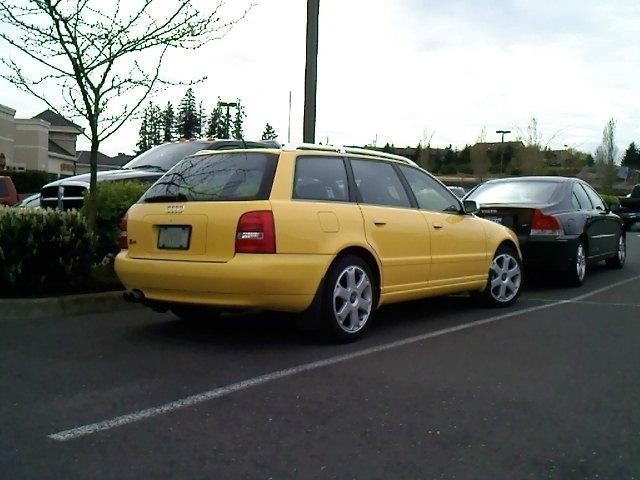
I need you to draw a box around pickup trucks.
[617,183,640,231]
[0,174,38,208]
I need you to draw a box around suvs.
[40,134,283,214]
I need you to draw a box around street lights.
[495,130,512,177]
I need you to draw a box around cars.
[457,172,632,294]
[447,186,467,201]
[13,192,41,210]
[114,142,527,348]
